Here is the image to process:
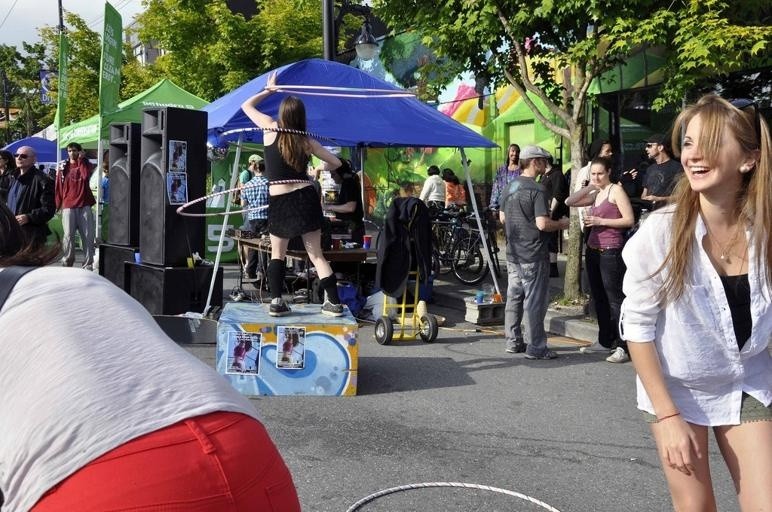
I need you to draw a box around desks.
[231,231,380,307]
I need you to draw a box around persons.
[319,159,364,248]
[567,156,635,365]
[282,332,298,366]
[621,96,772,512]
[442,169,468,214]
[171,142,186,169]
[233,340,250,371]
[487,143,522,247]
[537,156,565,277]
[563,131,638,235]
[170,175,182,203]
[418,166,447,215]
[239,70,343,317]
[240,162,268,278]
[239,154,264,273]
[498,144,569,361]
[0,141,109,269]
[638,133,688,209]
[0,203,302,512]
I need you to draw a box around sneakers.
[606,347,629,363]
[580,341,613,353]
[269,302,291,317]
[320,300,343,317]
[524,350,557,360]
[245,273,256,278]
[506,343,527,353]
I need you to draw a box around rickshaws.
[374,197,439,346]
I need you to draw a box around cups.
[332,238,340,249]
[135,253,140,263]
[363,235,372,249]
[476,291,484,304]
[495,294,501,302]
[187,257,194,268]
[582,209,592,215]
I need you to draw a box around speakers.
[123,261,223,315]
[107,122,140,247]
[139,108,208,266]
[98,244,139,295]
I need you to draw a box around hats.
[248,154,264,164]
[647,134,666,143]
[589,139,602,160]
[519,145,549,160]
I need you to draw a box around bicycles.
[425,201,501,285]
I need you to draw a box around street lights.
[321,0,379,63]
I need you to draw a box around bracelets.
[651,410,683,424]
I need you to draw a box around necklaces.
[702,210,746,260]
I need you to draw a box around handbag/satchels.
[232,178,241,204]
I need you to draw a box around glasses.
[14,154,28,159]
[645,144,652,147]
[727,98,761,146]
